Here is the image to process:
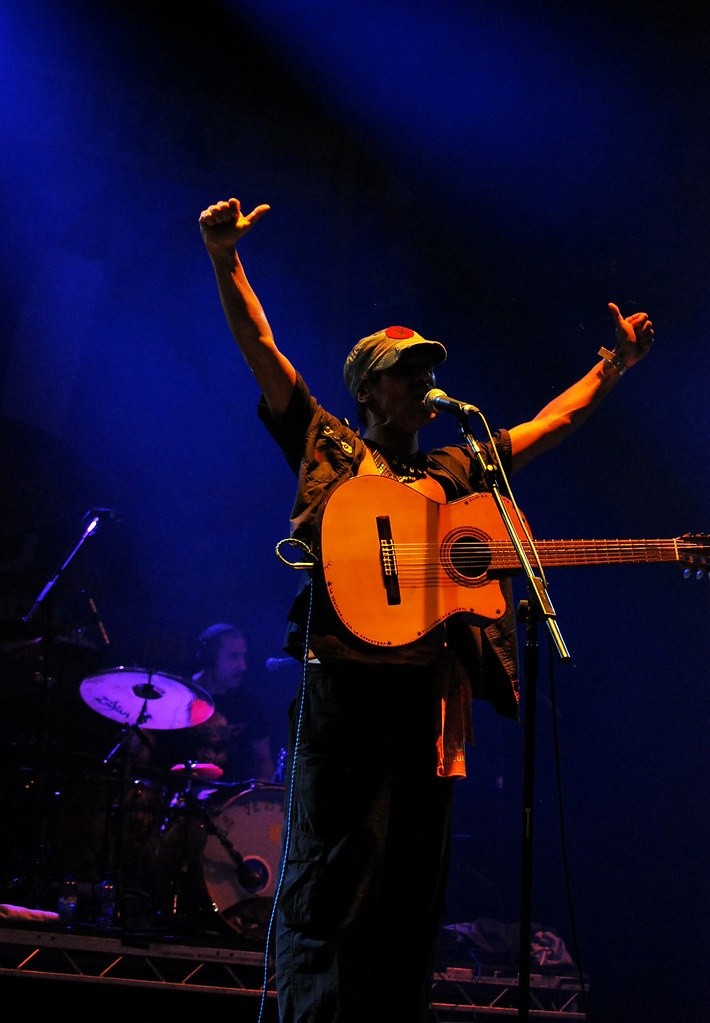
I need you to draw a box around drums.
[142,774,290,941]
[74,767,176,868]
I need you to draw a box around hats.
[343,326,447,397]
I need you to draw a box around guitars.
[309,475,710,655]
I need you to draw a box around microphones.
[93,508,124,521]
[266,658,299,672]
[425,389,480,417]
[246,870,262,883]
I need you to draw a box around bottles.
[95,872,115,930]
[58,876,80,929]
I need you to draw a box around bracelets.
[597,346,627,377]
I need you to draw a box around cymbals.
[78,665,216,731]
[1,617,125,670]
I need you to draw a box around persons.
[181,621,274,783]
[197,196,655,1023]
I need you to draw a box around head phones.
[195,626,238,672]
